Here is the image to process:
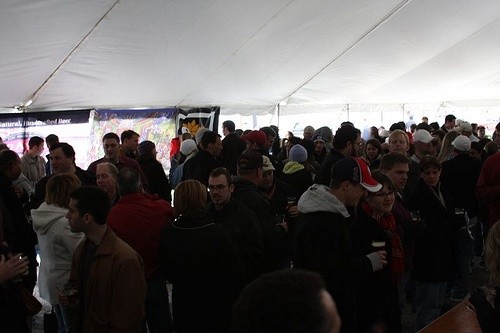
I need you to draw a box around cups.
[455,208,467,229]
[411,211,421,225]
[276,214,286,225]
[287,196,296,208]
[371,241,386,252]
[57,283,79,307]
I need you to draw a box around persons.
[203,166,265,308]
[377,152,436,333]
[346,170,407,333]
[57,186,147,333]
[105,166,176,333]
[467,220,500,333]
[25,143,96,209]
[289,157,387,333]
[0,114,500,291]
[159,178,235,333]
[30,172,86,333]
[0,210,43,333]
[412,154,468,316]
[229,269,342,333]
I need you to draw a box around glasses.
[208,184,230,190]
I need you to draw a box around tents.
[0,0,500,147]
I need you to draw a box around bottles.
[2,242,24,284]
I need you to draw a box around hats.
[452,121,481,143]
[451,135,471,151]
[329,156,383,193]
[312,135,324,143]
[179,139,199,156]
[289,144,308,162]
[196,128,211,145]
[379,129,390,137]
[260,155,276,171]
[413,129,435,143]
